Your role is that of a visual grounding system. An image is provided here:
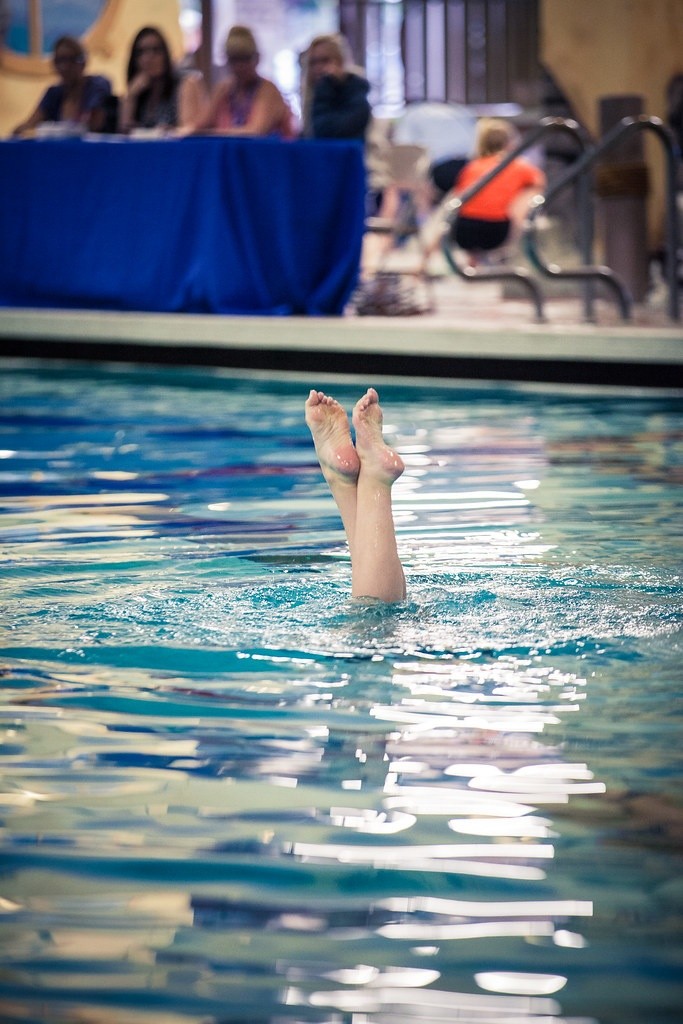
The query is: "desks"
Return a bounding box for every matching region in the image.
[0,133,369,318]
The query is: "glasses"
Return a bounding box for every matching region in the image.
[227,52,256,62]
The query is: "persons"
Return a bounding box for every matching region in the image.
[12,23,373,143]
[305,387,406,797]
[420,117,547,269]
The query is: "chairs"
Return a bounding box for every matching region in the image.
[366,145,426,278]
